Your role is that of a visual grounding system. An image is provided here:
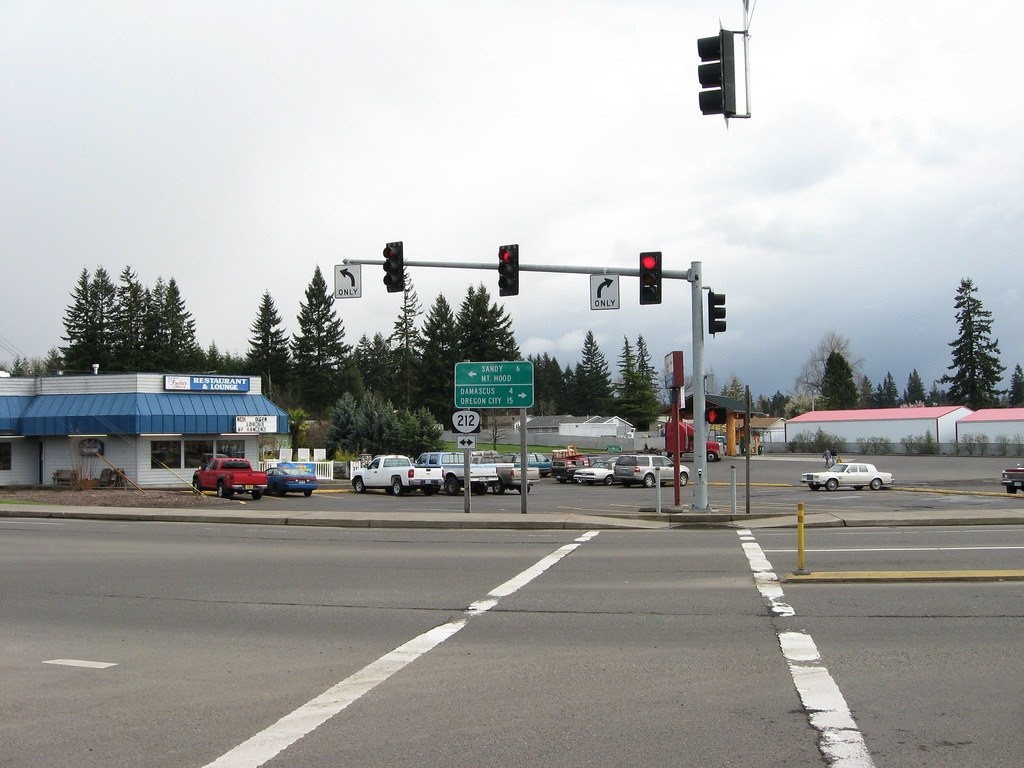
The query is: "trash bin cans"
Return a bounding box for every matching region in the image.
[741,447,746,454]
[607,445,623,453]
[758,445,763,455]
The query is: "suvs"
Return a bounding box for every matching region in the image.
[500,452,552,476]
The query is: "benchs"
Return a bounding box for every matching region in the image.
[52,469,78,486]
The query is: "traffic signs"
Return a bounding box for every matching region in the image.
[590,273,620,311]
[454,361,535,408]
[333,264,362,298]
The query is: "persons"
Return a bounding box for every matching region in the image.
[823,446,837,468]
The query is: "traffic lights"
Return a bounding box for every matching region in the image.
[639,251,662,305]
[707,292,727,334]
[697,30,737,116]
[382,241,404,293]
[498,244,519,297]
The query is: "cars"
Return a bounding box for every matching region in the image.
[573,461,617,486]
[613,454,690,488]
[1001,463,1024,494]
[265,467,318,497]
[800,463,893,492]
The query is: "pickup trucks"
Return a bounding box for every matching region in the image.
[412,452,498,496]
[188,453,230,467]
[351,454,445,497]
[193,458,268,501]
[551,456,606,484]
[471,451,541,495]
